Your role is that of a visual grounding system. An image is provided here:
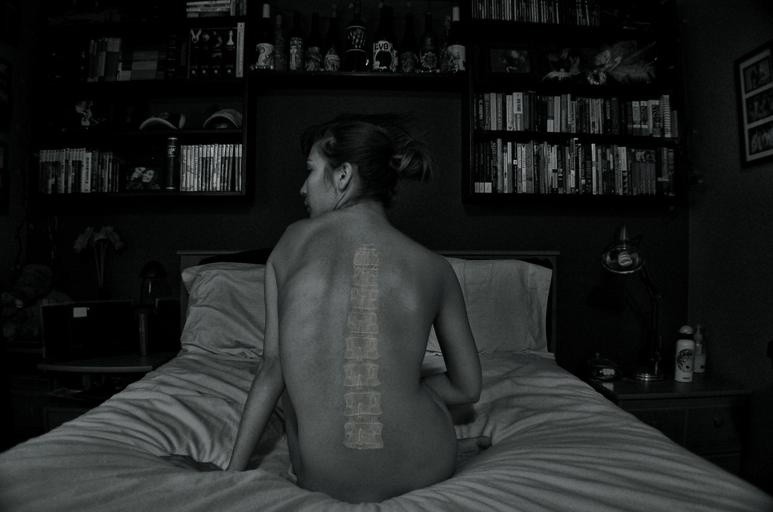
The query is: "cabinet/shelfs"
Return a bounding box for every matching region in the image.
[0,1,249,200]
[4,340,49,428]
[460,1,691,217]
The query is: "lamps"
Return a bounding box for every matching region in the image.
[599,224,665,383]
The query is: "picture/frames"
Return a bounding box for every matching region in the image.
[733,44,773,168]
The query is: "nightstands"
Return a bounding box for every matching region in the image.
[36,355,154,433]
[561,360,751,478]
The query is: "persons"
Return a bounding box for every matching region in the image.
[225,108,493,505]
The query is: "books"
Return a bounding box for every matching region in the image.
[474,88,680,197]
[33,146,121,194]
[87,37,122,83]
[179,144,242,193]
[470,0,592,28]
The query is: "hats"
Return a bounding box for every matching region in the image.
[202,108,242,129]
[140,109,186,130]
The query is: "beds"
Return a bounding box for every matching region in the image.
[0,248,772,512]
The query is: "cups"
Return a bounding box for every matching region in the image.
[287,36,304,69]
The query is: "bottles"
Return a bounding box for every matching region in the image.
[399,5,467,73]
[303,46,322,71]
[672,325,706,382]
[323,49,340,72]
[273,15,287,70]
[253,3,274,68]
